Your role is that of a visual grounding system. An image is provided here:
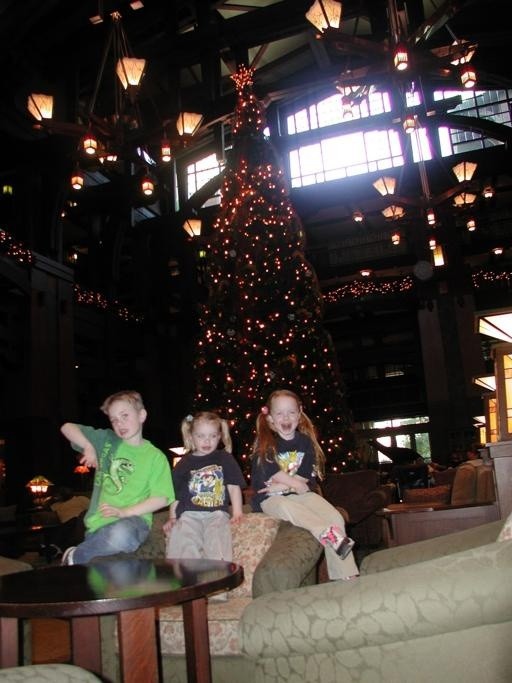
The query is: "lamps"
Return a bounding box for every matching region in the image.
[471,304,512,445]
[25,475,55,507]
[350,111,505,267]
[303,0,477,135]
[28,10,204,197]
[183,213,203,245]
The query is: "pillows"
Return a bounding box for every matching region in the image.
[158,513,281,593]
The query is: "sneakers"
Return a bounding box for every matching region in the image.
[60,546,77,567]
[319,527,356,561]
[208,592,229,603]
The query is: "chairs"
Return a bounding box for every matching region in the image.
[240,514,509,680]
[110,511,330,658]
[331,470,389,543]
[377,461,495,548]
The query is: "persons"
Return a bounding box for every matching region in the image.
[60,391,175,566]
[428,452,463,488]
[252,389,359,580]
[163,411,247,601]
